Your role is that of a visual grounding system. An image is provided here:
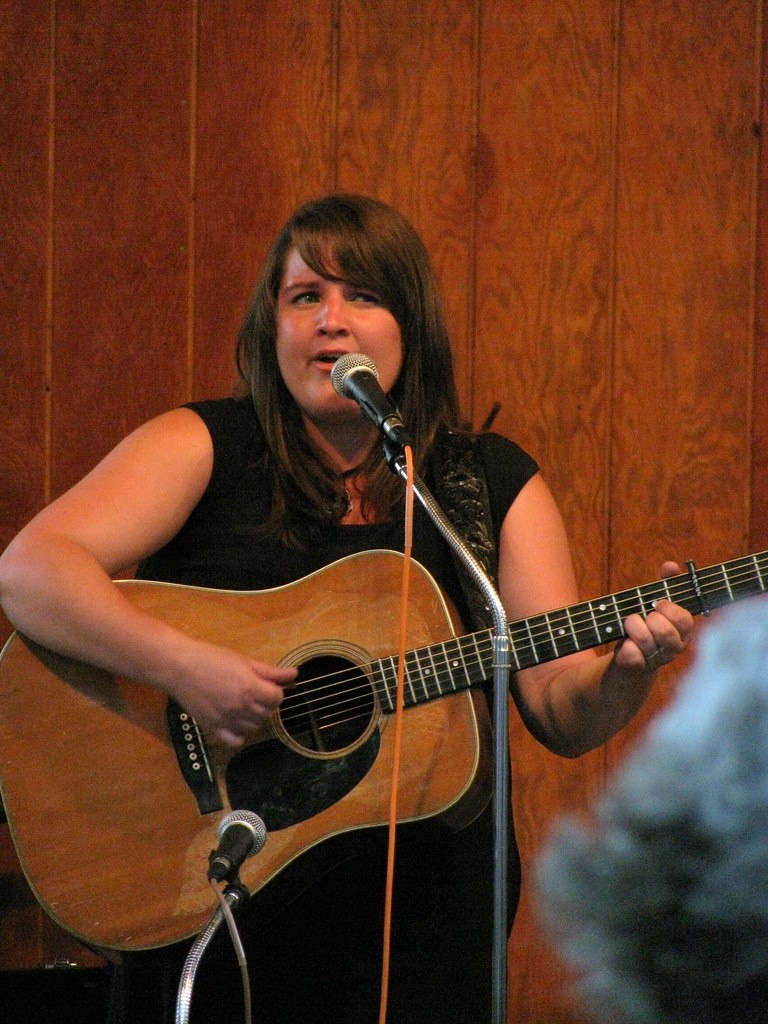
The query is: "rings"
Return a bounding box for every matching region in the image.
[645,651,659,659]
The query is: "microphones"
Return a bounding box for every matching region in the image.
[207,809,267,883]
[329,352,412,449]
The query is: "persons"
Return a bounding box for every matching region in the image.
[535,595,768,1023]
[1,191,694,1024]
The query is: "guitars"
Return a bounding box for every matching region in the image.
[0,549,768,955]
